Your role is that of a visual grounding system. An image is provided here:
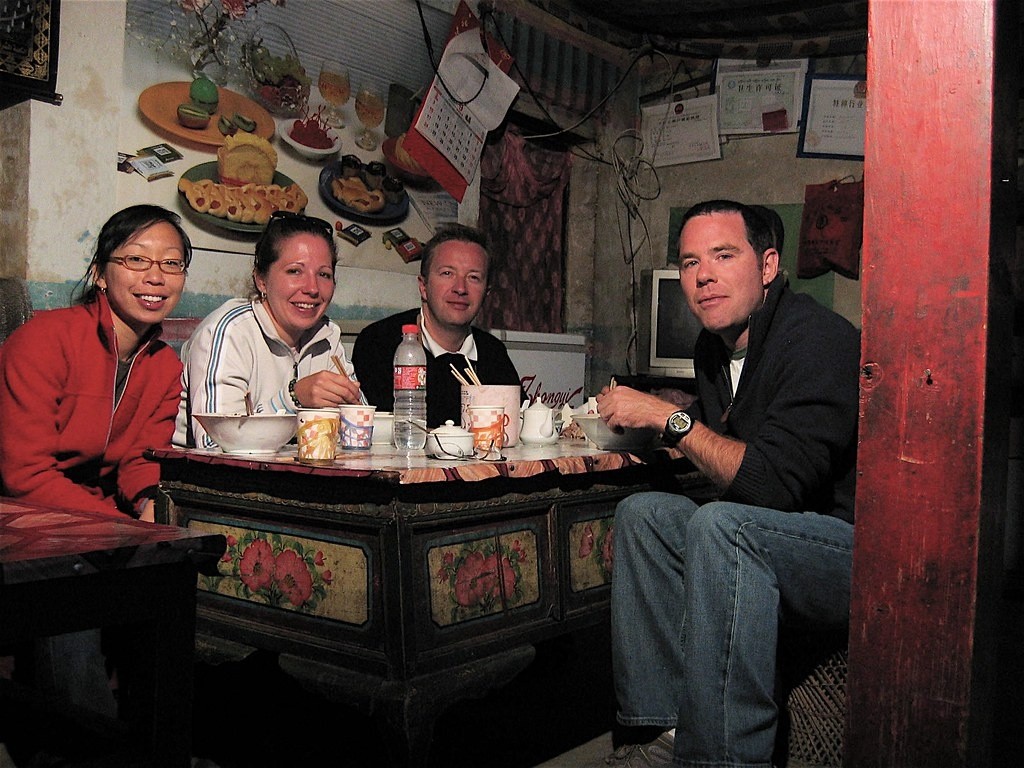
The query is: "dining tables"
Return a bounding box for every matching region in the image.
[138,438,725,768]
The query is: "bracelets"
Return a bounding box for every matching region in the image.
[289,378,300,407]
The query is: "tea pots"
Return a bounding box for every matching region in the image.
[519,397,558,445]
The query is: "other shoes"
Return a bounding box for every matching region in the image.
[584,729,676,768]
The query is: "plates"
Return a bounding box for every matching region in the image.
[382,138,434,180]
[177,160,306,232]
[319,160,409,221]
[139,82,276,145]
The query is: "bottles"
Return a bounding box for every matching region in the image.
[393,324,427,449]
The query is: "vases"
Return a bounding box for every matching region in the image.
[187,10,232,87]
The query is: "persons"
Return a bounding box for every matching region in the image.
[352,223,532,430]
[169,211,368,477]
[594,200,862,768]
[0,206,194,720]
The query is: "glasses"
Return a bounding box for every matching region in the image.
[255,210,335,253]
[103,254,189,275]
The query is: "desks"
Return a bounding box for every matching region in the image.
[0,497,225,768]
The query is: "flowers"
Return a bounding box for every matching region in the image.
[125,1,308,110]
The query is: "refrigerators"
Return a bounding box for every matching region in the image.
[329,319,587,411]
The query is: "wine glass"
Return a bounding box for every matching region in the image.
[353,82,385,152]
[318,60,351,128]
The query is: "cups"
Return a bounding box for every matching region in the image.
[467,405,505,450]
[296,408,341,465]
[460,385,521,447]
[338,405,376,450]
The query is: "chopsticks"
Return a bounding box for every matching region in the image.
[331,355,349,379]
[449,356,481,386]
[244,390,255,416]
[610,377,616,391]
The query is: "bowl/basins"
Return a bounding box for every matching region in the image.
[371,411,395,445]
[570,413,657,451]
[426,420,475,460]
[278,118,343,159]
[190,413,298,454]
[554,420,565,434]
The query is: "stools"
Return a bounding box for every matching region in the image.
[779,625,849,768]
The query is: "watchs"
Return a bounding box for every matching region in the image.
[663,409,697,446]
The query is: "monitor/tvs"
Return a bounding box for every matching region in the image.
[649,268,705,379]
